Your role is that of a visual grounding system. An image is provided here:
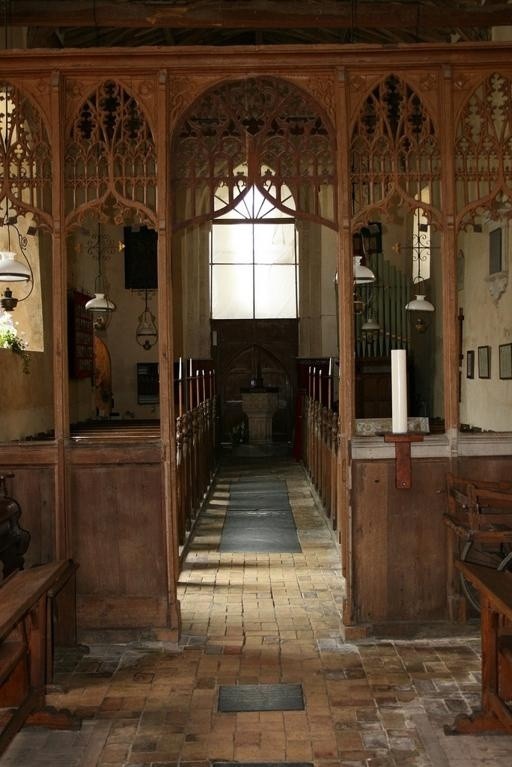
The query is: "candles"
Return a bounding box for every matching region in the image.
[389,349,410,435]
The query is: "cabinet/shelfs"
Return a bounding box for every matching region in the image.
[67,286,97,379]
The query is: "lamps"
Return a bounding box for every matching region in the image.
[335,210,436,336]
[85,223,116,318]
[0,91,33,300]
[135,291,159,350]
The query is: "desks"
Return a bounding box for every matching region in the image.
[443,560,511,739]
[2,558,85,765]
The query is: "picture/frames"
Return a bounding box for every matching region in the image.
[464,342,512,380]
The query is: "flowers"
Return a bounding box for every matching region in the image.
[0,314,33,375]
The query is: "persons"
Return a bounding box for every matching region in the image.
[354,366,371,418]
